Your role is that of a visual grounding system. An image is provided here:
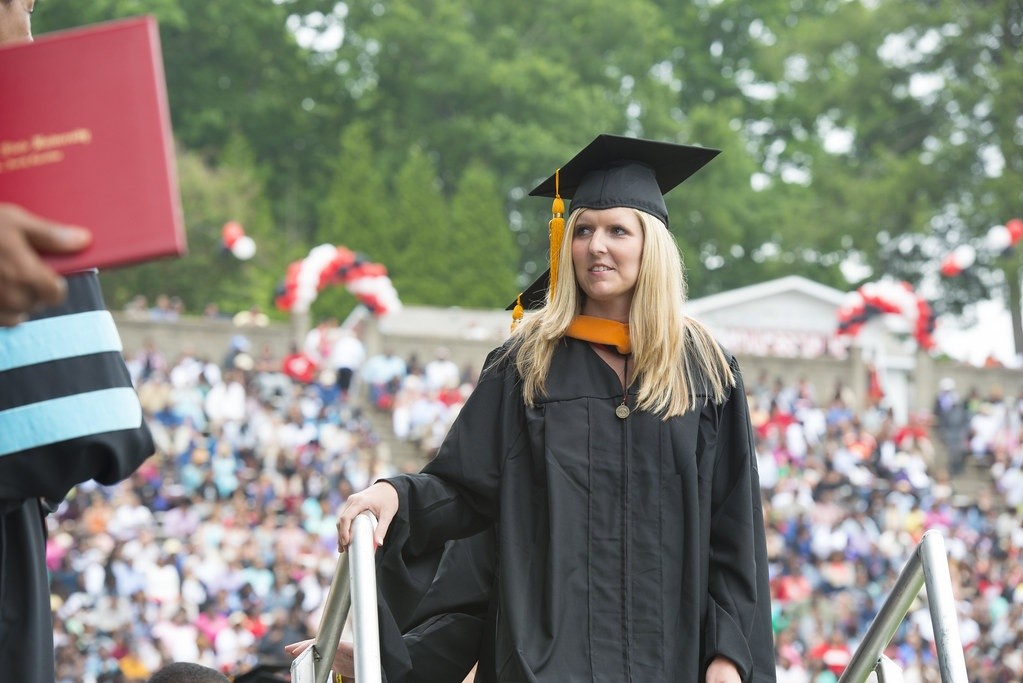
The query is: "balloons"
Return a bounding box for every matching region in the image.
[918,218,1023,300]
[272,245,402,317]
[221,222,256,261]
[835,276,934,350]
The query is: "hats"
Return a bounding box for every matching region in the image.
[528,133,724,313]
[506,264,552,335]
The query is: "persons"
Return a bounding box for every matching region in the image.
[0,0,157,683]
[44,296,483,683]
[738,376,1023,683]
[338,133,776,683]
